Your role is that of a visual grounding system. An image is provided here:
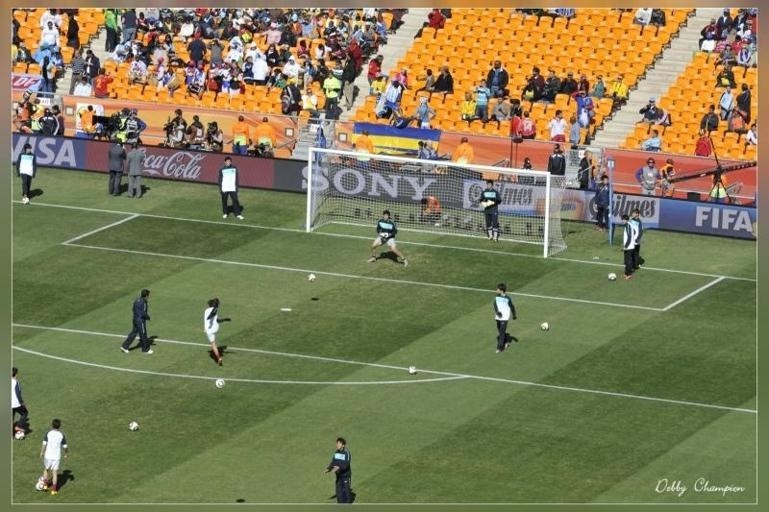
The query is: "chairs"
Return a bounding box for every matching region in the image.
[12,9,759,160]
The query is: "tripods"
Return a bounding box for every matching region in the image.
[704,179,731,204]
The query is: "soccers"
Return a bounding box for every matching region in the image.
[308,273,315,282]
[409,366,416,373]
[129,421,138,431]
[23,198,30,205]
[216,379,225,388]
[608,273,616,281]
[15,431,24,440]
[36,482,45,491]
[541,322,548,330]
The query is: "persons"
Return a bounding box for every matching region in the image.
[620,215,635,279]
[120,288,154,354]
[367,209,409,267]
[493,282,517,353]
[630,208,646,270]
[40,418,69,494]
[12,8,758,243]
[203,297,232,367]
[324,437,353,503]
[12,367,29,432]
